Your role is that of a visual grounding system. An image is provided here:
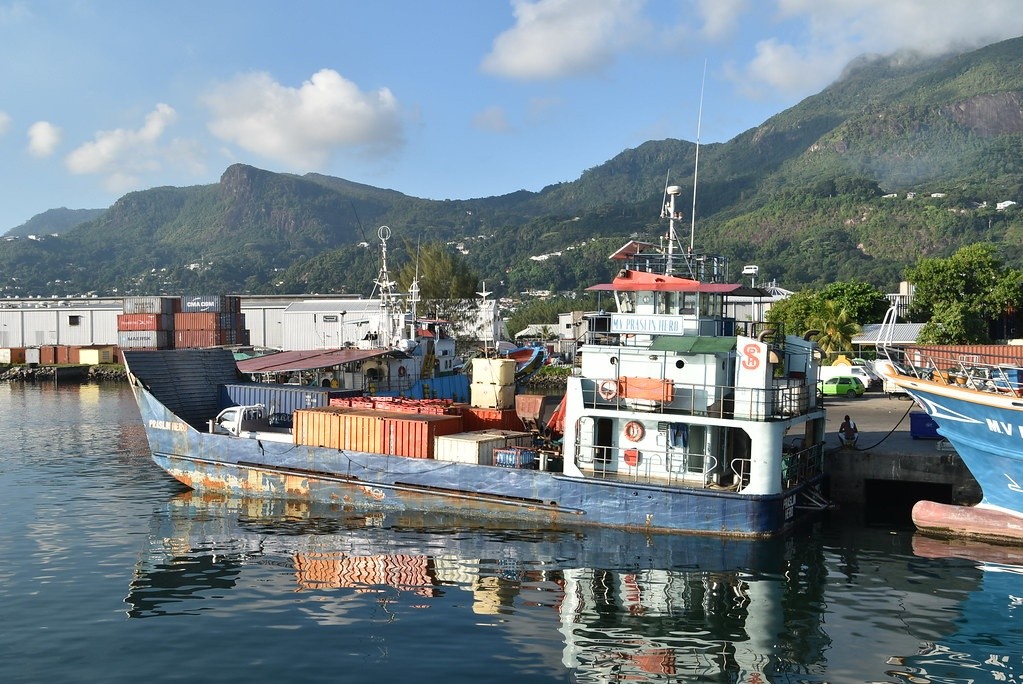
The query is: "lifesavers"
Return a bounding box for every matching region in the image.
[624,422,644,440]
[600,380,618,400]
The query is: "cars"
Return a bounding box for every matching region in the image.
[816,364,881,399]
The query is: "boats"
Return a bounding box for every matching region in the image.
[122,170,837,541]
[862,293,1022,517]
[240,224,547,405]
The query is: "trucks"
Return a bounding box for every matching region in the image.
[205,403,293,444]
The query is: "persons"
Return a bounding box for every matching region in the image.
[838,415,859,448]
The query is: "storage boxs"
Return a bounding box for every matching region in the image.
[903,344,1022,392]
[0,293,546,470]
